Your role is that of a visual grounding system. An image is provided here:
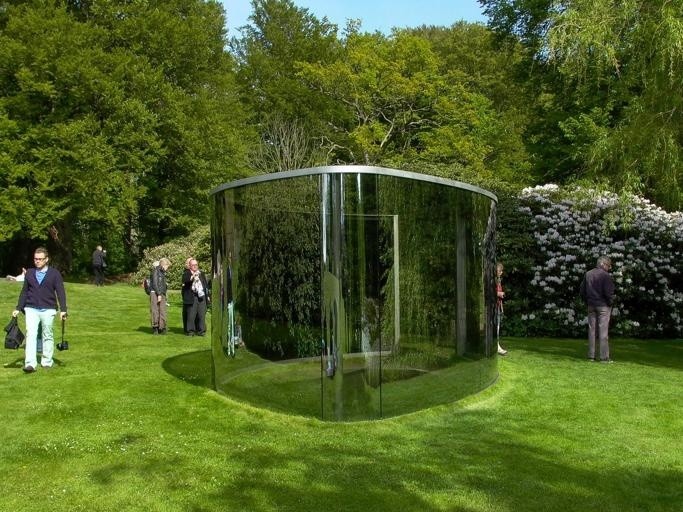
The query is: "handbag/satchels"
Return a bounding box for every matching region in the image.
[5,325,25,349]
[144,279,150,294]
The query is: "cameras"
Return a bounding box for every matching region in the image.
[57,340,69,351]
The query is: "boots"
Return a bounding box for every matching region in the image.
[154,327,167,335]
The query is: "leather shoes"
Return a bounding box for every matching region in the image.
[23,366,36,372]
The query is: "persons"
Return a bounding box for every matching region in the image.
[211,249,245,358]
[148,257,171,335]
[182,259,211,337]
[10,246,66,373]
[579,255,617,365]
[486,262,508,355]
[180,257,202,333]
[91,245,107,287]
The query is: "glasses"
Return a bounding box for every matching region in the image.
[34,258,46,261]
